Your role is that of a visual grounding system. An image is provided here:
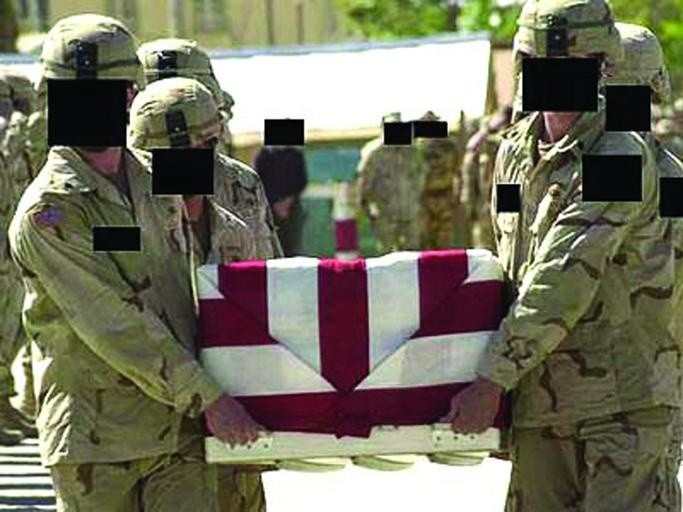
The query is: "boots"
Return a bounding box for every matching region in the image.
[0,395,38,444]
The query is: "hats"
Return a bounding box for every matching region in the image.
[380,111,402,123]
[421,111,437,121]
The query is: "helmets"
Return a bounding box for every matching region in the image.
[129,40,234,150]
[0,75,34,103]
[511,0,671,105]
[39,13,146,84]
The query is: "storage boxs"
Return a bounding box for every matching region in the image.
[196,247,514,464]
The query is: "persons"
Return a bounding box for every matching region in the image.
[598,22,681,268]
[127,78,268,512]
[437,1,680,511]
[0,39,306,448]
[7,13,268,512]
[359,106,509,255]
[650,100,682,164]
